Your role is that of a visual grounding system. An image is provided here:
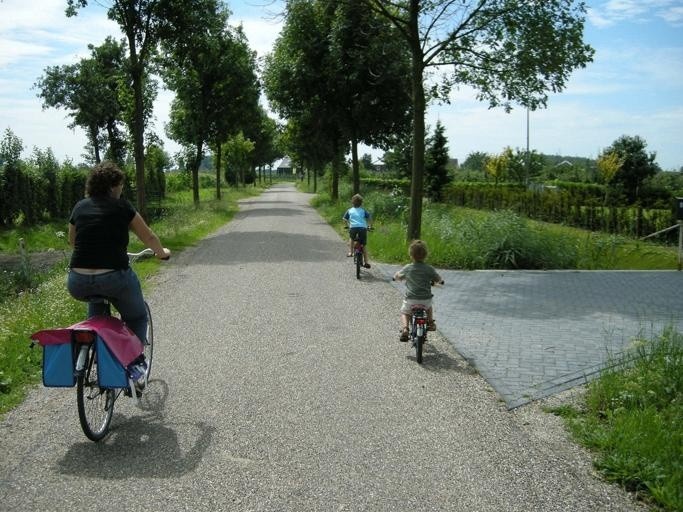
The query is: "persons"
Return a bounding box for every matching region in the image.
[67,159,170,389]
[343,193,371,270]
[395,240,442,341]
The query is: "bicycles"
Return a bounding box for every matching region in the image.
[391,276,445,364]
[76,249,170,443]
[344,226,375,279]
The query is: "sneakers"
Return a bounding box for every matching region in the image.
[130,360,147,382]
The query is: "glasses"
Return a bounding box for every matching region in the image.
[119,183,123,188]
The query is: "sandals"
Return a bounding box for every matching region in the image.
[347,251,353,257]
[364,263,370,268]
[427,321,436,331]
[399,327,410,342]
